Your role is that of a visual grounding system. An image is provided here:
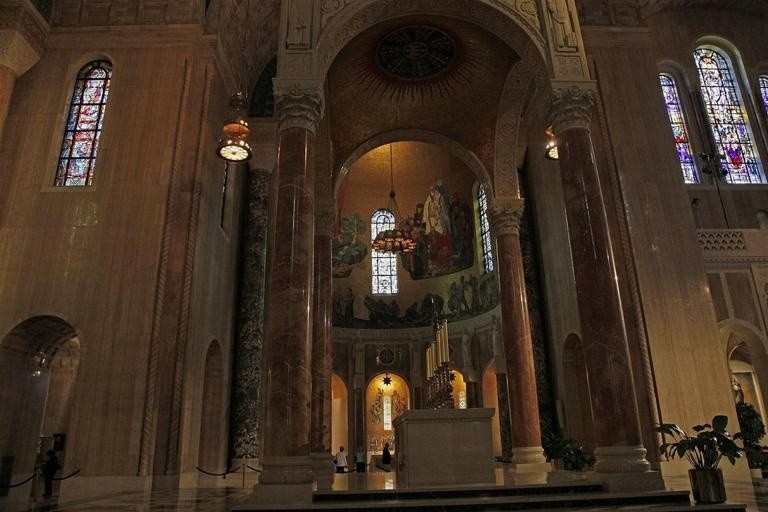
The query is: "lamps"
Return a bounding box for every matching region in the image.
[545,120,560,162]
[219,0,253,165]
[369,144,417,256]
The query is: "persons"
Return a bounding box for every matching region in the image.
[398,175,476,282]
[381,442,392,472]
[41,449,58,498]
[353,446,366,473]
[334,446,348,473]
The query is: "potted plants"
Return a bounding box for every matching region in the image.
[653,399,767,503]
[541,417,596,479]
